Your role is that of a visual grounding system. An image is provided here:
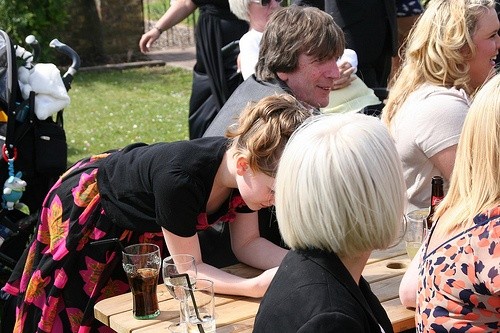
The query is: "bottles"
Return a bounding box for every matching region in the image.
[427,175,445,234]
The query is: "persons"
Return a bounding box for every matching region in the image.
[229,0,384,119]
[201,4,345,269]
[254,113,408,333]
[296,0,398,88]
[379,0,500,212]
[140,0,229,138]
[399,74,500,333]
[0,93,312,333]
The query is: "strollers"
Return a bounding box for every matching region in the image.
[0,28,82,333]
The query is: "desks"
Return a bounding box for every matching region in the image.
[93,226,416,332]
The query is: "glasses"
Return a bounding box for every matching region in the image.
[250,0,282,7]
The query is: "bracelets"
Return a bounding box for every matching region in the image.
[153,26,162,34]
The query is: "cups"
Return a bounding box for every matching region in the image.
[183,279,216,333]
[122,243,162,319]
[404,208,430,260]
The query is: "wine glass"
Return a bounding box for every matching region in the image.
[162,254,198,333]
